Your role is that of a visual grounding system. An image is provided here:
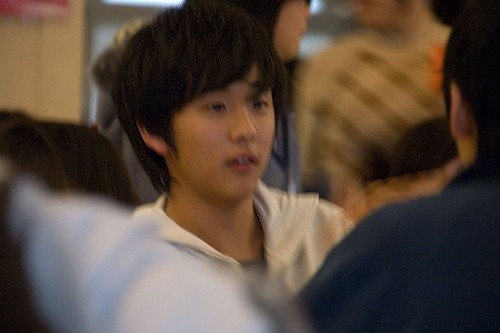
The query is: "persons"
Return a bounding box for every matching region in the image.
[0,0,461,225]
[287,0,500,333]
[108,0,358,333]
[0,157,270,333]
